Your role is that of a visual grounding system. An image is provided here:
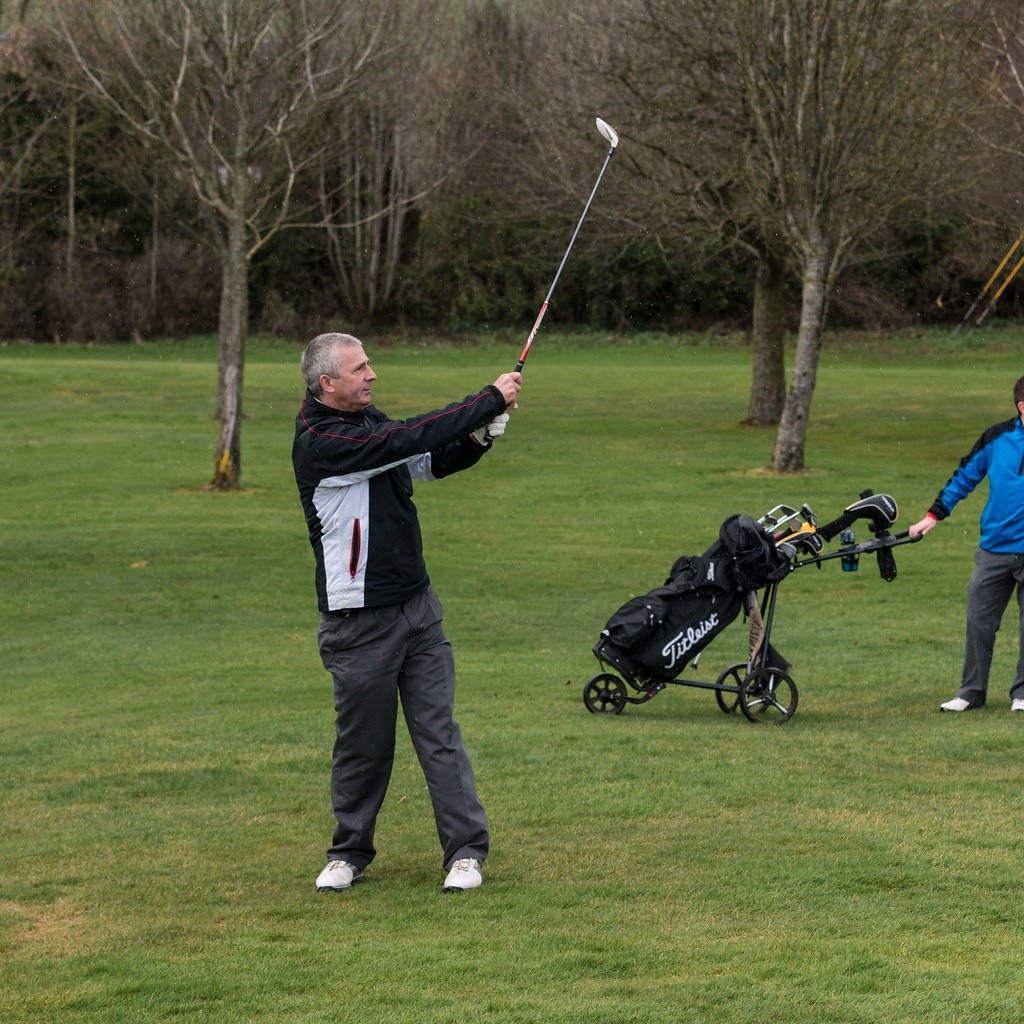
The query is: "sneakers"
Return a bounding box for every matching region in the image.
[442,857,482,891]
[940,697,977,711]
[1011,698,1024,711]
[315,858,363,890]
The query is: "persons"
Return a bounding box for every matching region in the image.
[293,333,523,894]
[908,375,1024,712]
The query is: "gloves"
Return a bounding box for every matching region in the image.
[472,403,518,446]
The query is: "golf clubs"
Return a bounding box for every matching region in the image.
[484,116,620,441]
[755,503,824,563]
